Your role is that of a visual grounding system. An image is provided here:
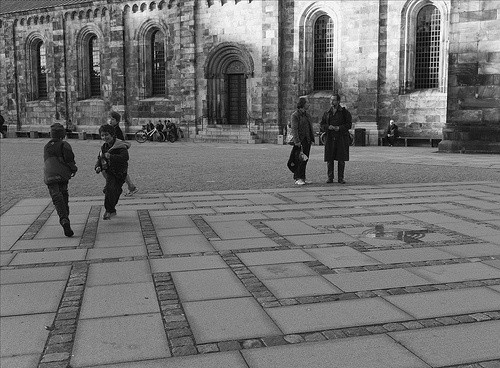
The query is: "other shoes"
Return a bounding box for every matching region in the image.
[326,179,333,182]
[125,188,139,196]
[102,211,116,220]
[338,180,345,184]
[62,221,74,237]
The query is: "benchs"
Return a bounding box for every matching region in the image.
[15,130,50,138]
[65,132,100,140]
[126,132,148,141]
[380,137,442,148]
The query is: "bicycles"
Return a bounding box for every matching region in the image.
[319,131,355,146]
[135,125,165,143]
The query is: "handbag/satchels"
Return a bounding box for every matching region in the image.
[287,145,303,173]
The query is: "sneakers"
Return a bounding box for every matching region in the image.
[295,179,305,184]
[305,180,312,183]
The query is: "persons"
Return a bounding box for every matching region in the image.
[386,120,399,147]
[320,94,352,184]
[291,97,315,185]
[145,119,181,142]
[94,124,131,220]
[44,123,78,238]
[108,111,139,196]
